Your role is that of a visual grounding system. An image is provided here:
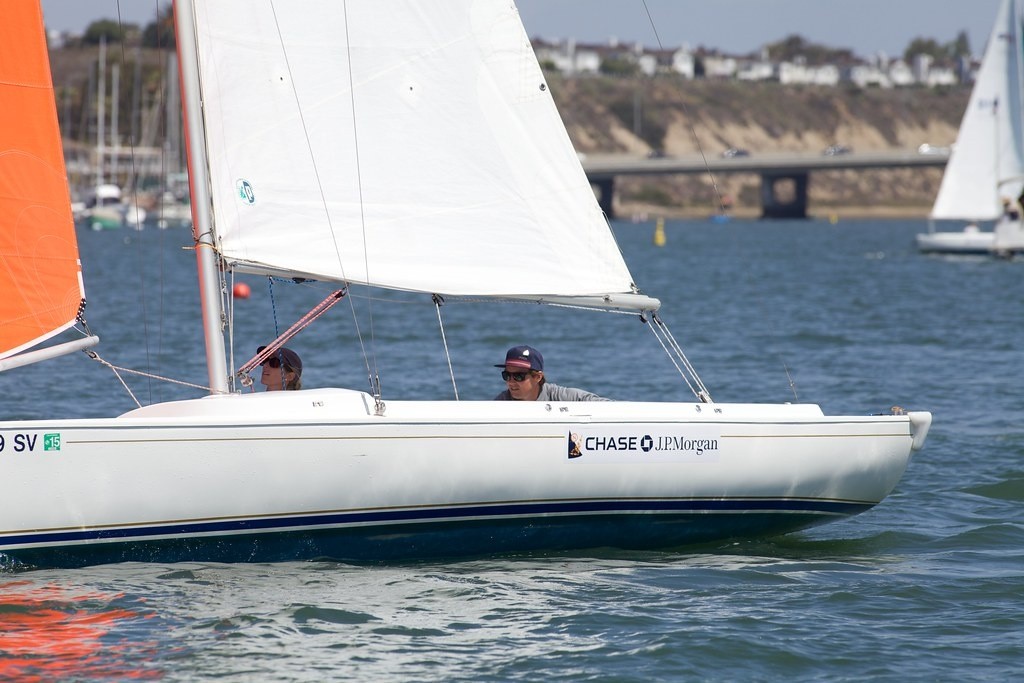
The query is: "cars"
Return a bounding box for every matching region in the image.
[645,148,673,159]
[719,145,751,157]
[821,142,854,157]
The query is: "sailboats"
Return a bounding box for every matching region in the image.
[917,0,1024,257]
[0,0,931,569]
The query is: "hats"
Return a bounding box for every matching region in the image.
[257,346,302,378]
[493,345,544,372]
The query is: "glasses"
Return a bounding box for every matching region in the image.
[259,354,281,368]
[501,371,527,382]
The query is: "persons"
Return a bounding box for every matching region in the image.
[256,346,302,391]
[492,345,614,401]
